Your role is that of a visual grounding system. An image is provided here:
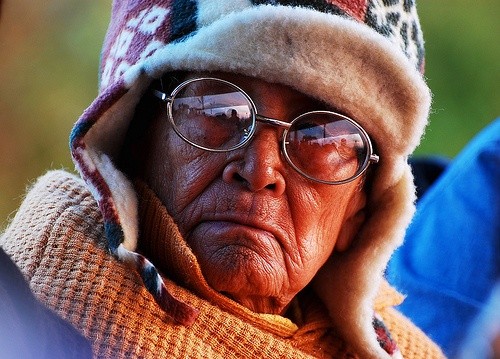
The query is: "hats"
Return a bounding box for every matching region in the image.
[69,2,434,359]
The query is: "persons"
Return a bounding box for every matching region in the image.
[0,1,447,359]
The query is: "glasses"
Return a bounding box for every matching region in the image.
[152,78,380,185]
